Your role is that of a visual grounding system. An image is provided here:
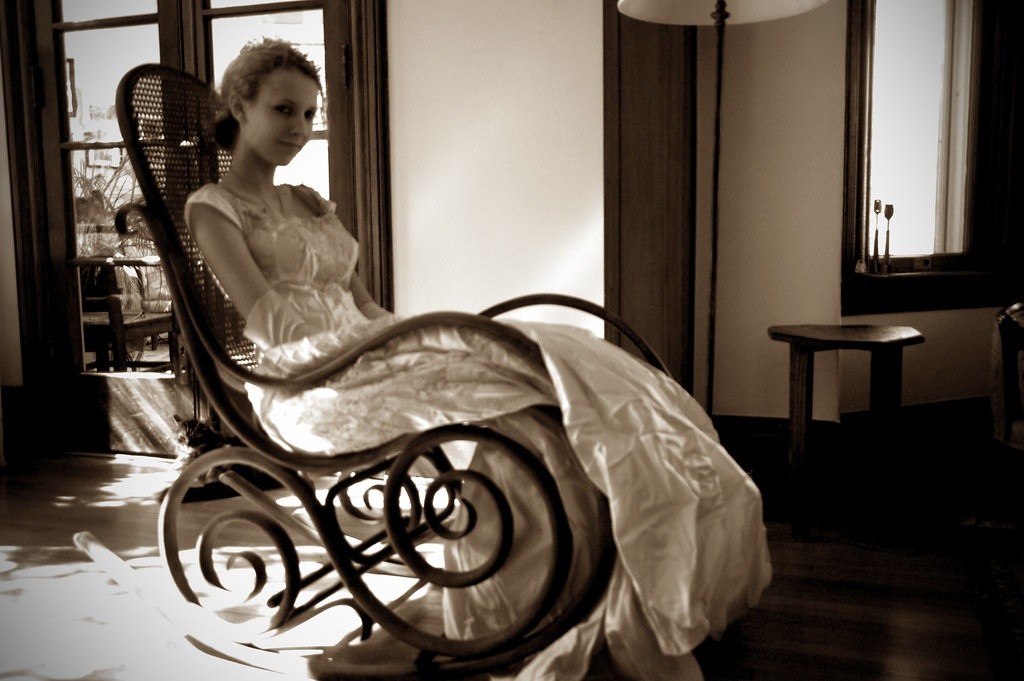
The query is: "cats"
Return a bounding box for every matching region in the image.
[153,414,286,506]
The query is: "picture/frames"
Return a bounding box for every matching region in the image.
[85,146,124,170]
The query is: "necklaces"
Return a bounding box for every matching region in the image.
[226,165,288,223]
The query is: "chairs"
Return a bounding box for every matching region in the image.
[73,63,676,681]
[73,254,185,373]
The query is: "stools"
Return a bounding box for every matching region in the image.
[767,325,925,543]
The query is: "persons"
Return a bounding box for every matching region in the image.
[184,37,773,681]
